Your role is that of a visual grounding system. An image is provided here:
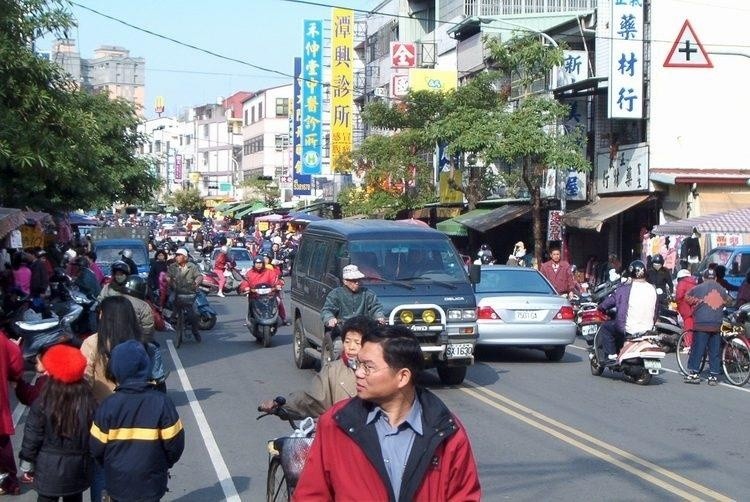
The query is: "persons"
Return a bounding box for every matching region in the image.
[291,327,482,502]
[509,241,750,386]
[0,215,103,327]
[89,339,185,502]
[258,313,379,420]
[102,214,303,276]
[1,296,142,493]
[91,248,146,311]
[360,247,438,279]
[20,345,100,502]
[320,264,389,358]
[148,249,202,341]
[214,244,228,298]
[239,256,292,326]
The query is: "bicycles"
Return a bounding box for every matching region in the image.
[674,306,749,389]
[251,395,318,501]
[170,280,199,348]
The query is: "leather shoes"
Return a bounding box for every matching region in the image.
[599,360,616,365]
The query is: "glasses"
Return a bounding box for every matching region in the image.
[356,360,388,372]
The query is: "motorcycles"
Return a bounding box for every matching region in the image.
[7,280,104,368]
[230,266,289,347]
[571,274,682,384]
[152,280,220,332]
[196,258,250,297]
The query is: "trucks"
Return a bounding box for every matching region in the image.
[693,244,749,308]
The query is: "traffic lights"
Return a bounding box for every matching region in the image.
[256,175,276,182]
[208,184,220,189]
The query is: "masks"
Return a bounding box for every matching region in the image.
[653,264,662,270]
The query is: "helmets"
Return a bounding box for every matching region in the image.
[123,249,132,259]
[119,275,145,298]
[253,256,263,262]
[630,261,644,278]
[111,260,129,273]
[652,254,663,262]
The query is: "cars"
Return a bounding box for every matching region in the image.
[465,262,578,360]
[75,208,292,282]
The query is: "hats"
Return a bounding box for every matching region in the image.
[703,268,716,279]
[677,269,691,279]
[176,249,187,256]
[343,264,364,279]
[41,344,87,383]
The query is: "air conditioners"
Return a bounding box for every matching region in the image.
[464,150,488,167]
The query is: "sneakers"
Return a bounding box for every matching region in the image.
[708,376,717,385]
[685,374,699,383]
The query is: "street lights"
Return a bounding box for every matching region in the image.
[477,14,567,260]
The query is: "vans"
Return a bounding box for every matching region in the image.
[283,219,479,385]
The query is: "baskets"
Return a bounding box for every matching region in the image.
[280,437,314,485]
[175,292,199,305]
